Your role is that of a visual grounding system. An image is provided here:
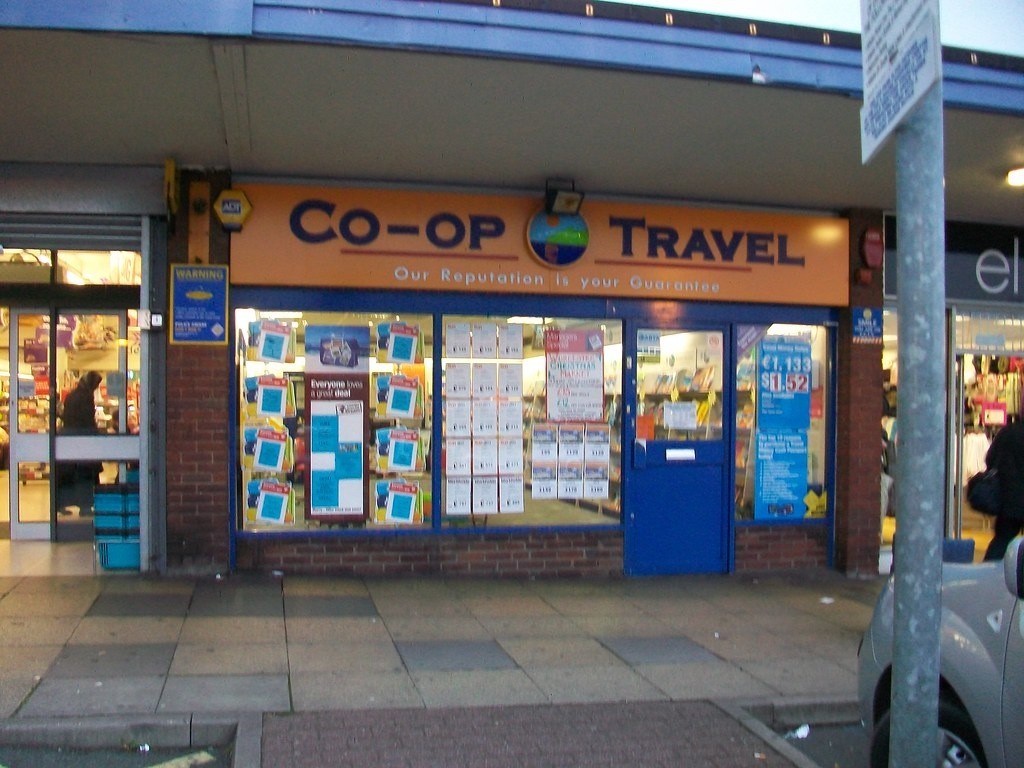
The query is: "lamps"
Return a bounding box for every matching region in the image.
[545,188,585,216]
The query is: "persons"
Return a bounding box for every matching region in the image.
[109,410,136,470]
[54,370,104,516]
[966,418,1024,563]
[879,385,898,473]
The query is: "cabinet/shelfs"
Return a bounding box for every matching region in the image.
[429,365,756,516]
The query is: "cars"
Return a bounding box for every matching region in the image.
[855,528,1024,768]
